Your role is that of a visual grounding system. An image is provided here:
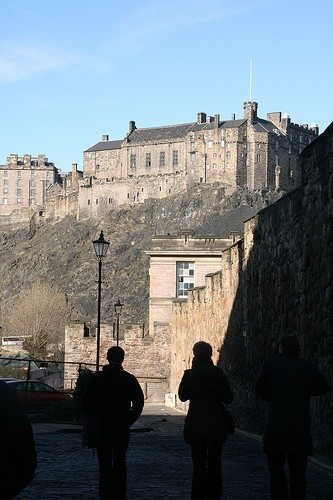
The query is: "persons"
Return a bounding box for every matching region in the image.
[76,347,144,500]
[255,336,330,500]
[0,378,40,500]
[178,341,236,500]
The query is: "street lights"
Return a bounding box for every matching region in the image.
[91,228,111,370]
[113,299,124,347]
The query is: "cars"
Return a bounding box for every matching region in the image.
[0,377,75,418]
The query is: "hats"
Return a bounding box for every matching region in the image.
[193,341,212,357]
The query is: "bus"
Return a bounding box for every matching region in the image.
[1,335,36,347]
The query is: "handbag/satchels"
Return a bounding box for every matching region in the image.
[209,411,232,446]
